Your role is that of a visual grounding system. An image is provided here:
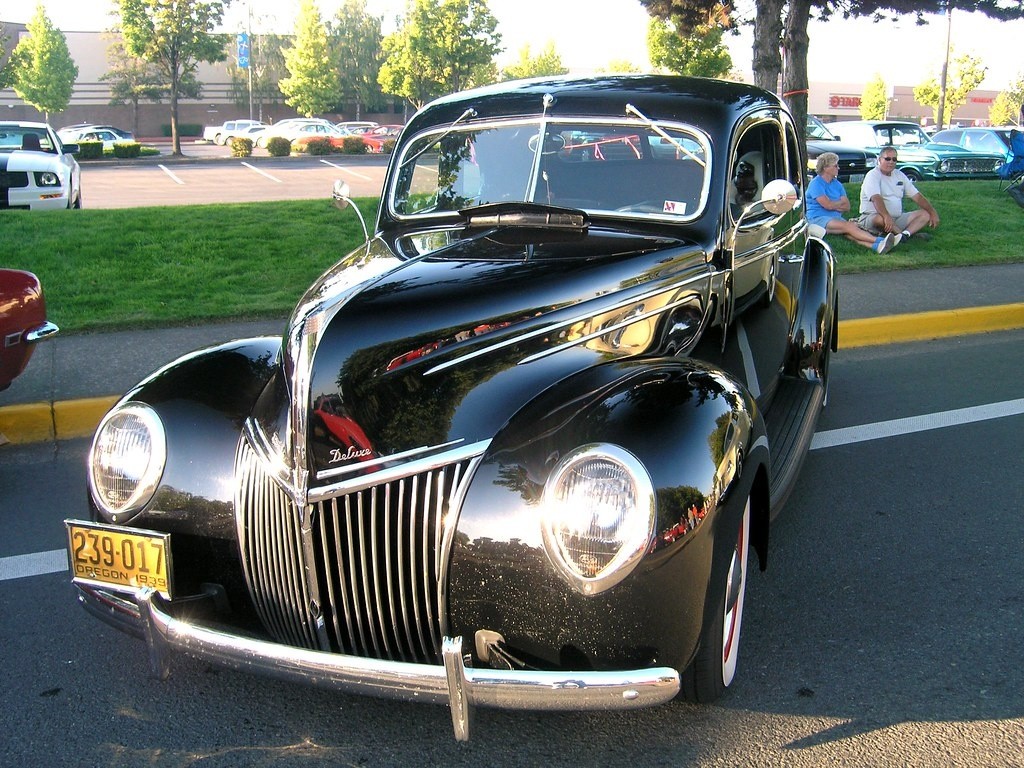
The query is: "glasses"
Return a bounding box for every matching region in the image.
[883,156,897,162]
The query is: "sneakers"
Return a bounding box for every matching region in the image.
[877,233,902,255]
[900,232,909,243]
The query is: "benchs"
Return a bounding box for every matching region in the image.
[524,158,706,216]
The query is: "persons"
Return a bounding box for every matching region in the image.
[805,152,894,255]
[858,147,939,243]
[685,504,700,530]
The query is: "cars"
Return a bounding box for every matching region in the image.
[0,267,60,392]
[62,73,841,743]
[55,124,135,150]
[0,121,81,211]
[806,114,1024,183]
[202,118,405,153]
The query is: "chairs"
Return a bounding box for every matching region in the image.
[995,129,1024,191]
